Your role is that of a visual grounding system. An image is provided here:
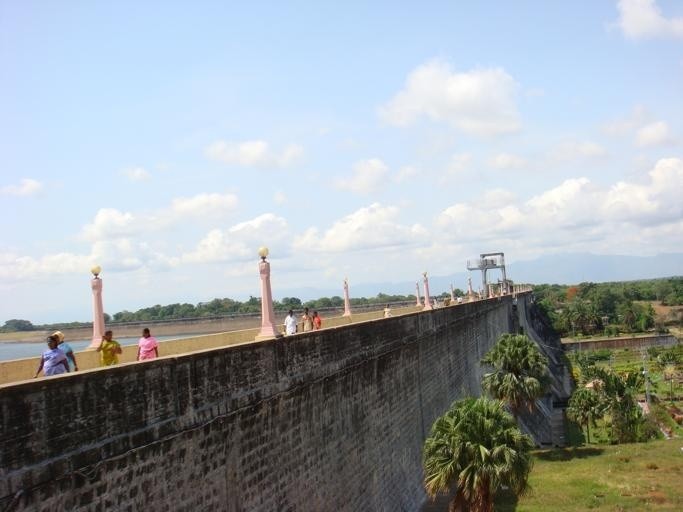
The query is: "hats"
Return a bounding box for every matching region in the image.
[52,331,64,343]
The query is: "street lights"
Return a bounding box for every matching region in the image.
[422,270,433,310]
[89,264,105,347]
[642,355,650,401]
[415,281,420,305]
[450,277,526,301]
[257,247,277,336]
[671,374,674,403]
[343,275,352,315]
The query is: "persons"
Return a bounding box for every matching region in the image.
[96,331,122,367]
[383,303,391,318]
[301,308,313,332]
[33,331,78,378]
[434,295,463,306]
[284,310,298,335]
[136,328,158,361]
[312,312,321,329]
